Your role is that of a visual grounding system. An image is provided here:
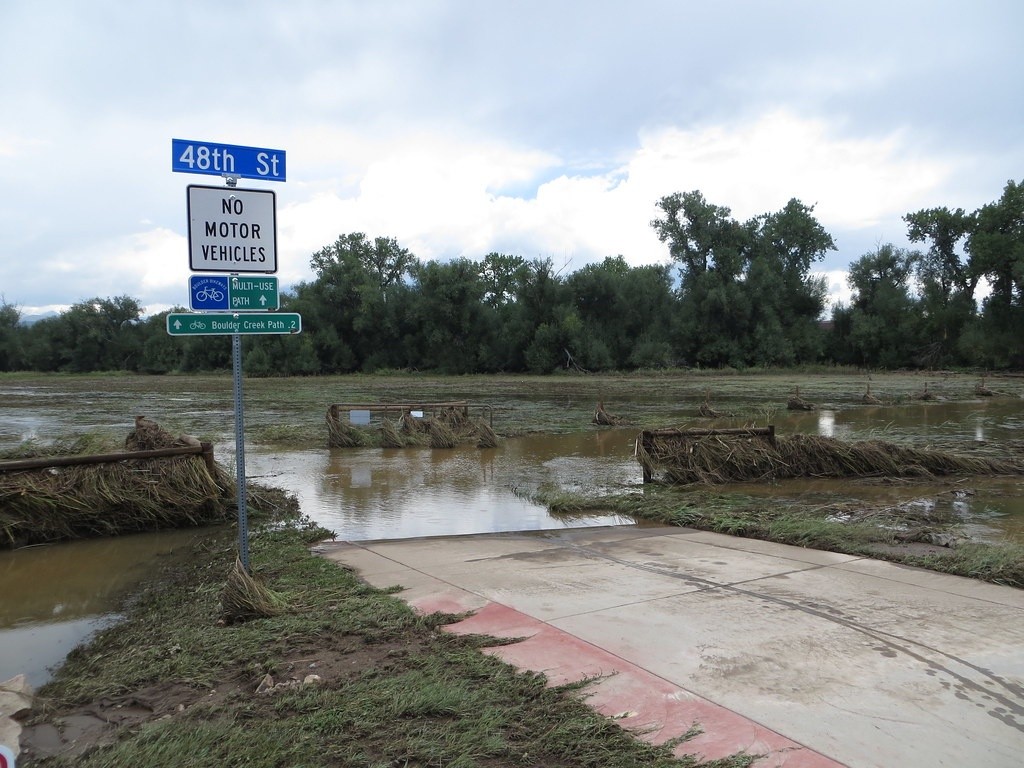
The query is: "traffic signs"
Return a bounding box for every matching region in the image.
[229,274,280,312]
[166,313,302,335]
